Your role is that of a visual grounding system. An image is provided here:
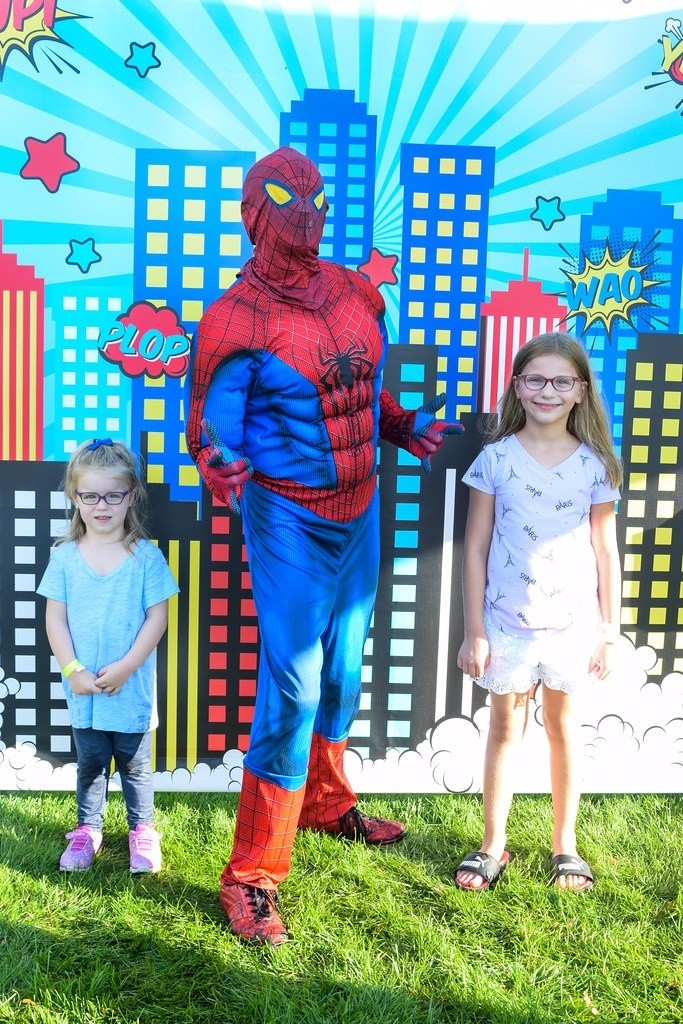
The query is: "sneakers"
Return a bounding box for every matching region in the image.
[128,824,162,874]
[320,805,408,845]
[60,825,102,870]
[218,882,288,945]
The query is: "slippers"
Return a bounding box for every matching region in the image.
[454,847,510,891]
[547,849,596,894]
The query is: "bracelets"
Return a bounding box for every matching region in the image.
[61,660,85,677]
[604,641,615,645]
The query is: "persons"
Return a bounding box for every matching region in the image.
[455,333,623,890]
[187,149,465,946]
[36,439,181,871]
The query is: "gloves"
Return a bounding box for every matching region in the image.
[410,393,465,472]
[196,419,253,514]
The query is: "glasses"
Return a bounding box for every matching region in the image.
[516,373,581,392]
[75,489,133,505]
